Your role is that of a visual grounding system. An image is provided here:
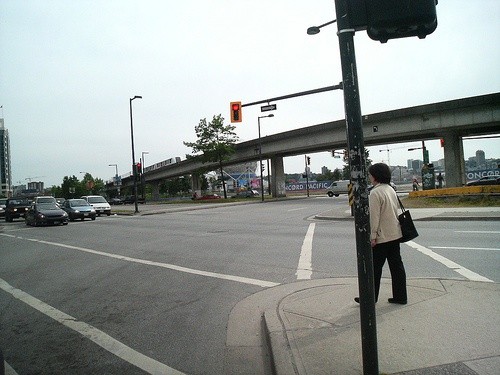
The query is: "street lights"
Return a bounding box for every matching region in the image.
[258,113,274,201]
[129,96,143,213]
[408,147,425,166]
[142,151,149,204]
[109,164,120,198]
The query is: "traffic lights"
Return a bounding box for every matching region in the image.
[229,102,242,122]
[261,164,265,171]
[307,157,310,165]
[332,149,335,157]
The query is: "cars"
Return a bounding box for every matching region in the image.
[56,198,65,205]
[32,196,60,207]
[108,198,123,205]
[81,196,111,216]
[465,175,500,185]
[24,203,69,227]
[0,198,33,222]
[61,199,96,222]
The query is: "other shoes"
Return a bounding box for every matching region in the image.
[354,297,360,303]
[388,298,408,304]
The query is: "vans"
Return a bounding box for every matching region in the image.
[325,180,350,198]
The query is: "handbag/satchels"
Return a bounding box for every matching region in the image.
[398,210,418,243]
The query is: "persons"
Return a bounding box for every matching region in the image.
[436,173,444,189]
[354,163,407,305]
[413,179,420,191]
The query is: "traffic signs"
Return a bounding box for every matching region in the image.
[261,104,276,111]
